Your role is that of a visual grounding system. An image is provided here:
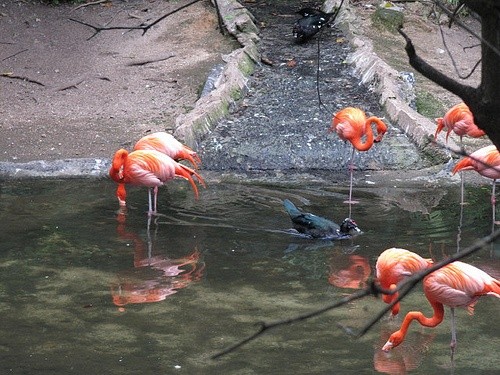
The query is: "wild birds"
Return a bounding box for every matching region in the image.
[381,260,500,352]
[111,206,206,306]
[373,247,434,317]
[373,317,438,375]
[432,102,486,146]
[329,202,371,290]
[108,132,206,211]
[450,226,500,281]
[327,107,388,204]
[451,144,500,225]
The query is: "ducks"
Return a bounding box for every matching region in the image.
[284,197,362,239]
[283,239,360,256]
